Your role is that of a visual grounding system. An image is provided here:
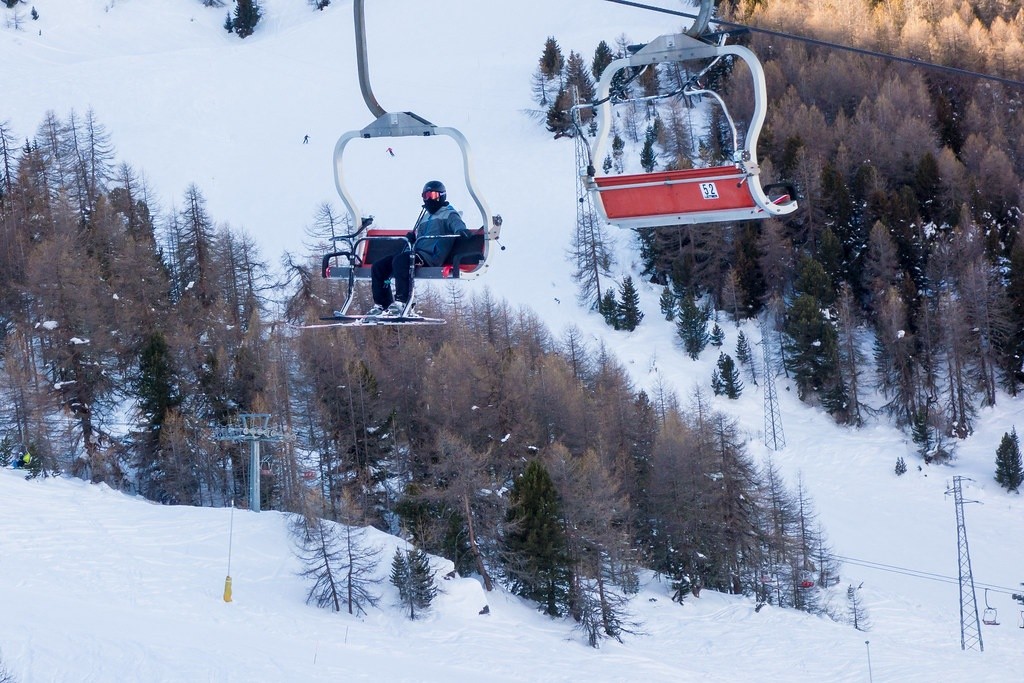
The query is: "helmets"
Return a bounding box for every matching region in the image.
[422,181,446,205]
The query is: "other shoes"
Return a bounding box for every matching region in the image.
[382,300,414,316]
[361,304,383,322]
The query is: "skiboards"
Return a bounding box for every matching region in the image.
[284,309,448,330]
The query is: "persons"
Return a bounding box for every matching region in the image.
[13,451,31,468]
[360,181,473,322]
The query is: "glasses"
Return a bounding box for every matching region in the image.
[423,191,440,200]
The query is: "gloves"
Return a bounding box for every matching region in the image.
[460,229,472,241]
[406,232,416,244]
